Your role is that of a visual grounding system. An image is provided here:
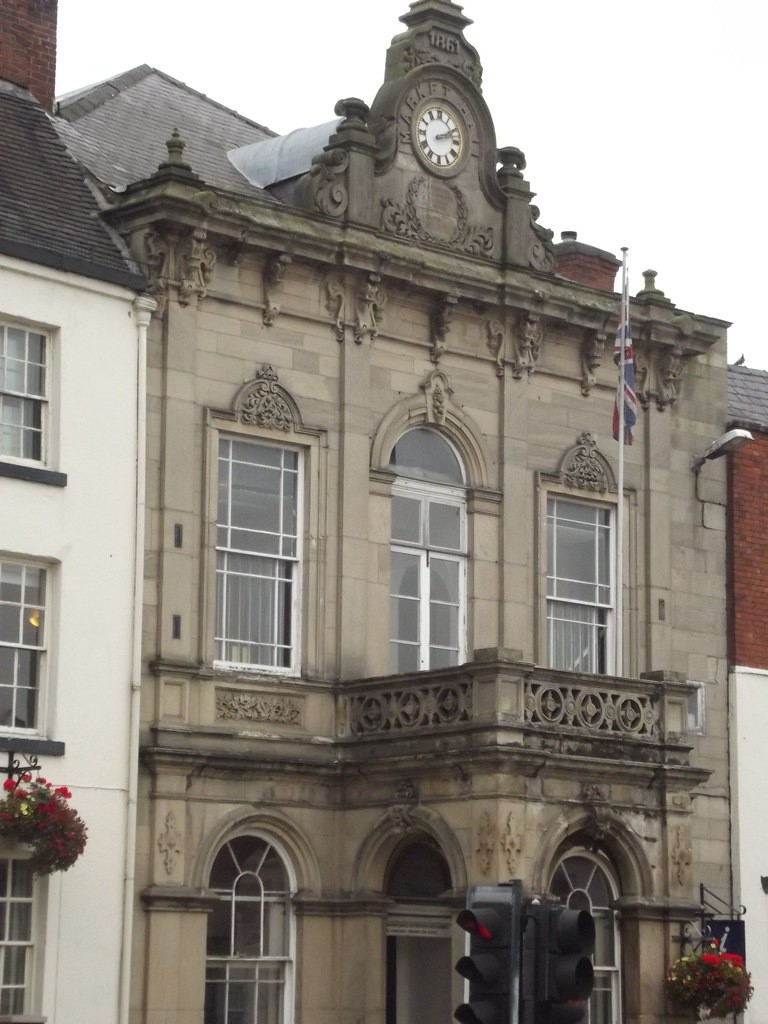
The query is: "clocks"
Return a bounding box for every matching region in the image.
[410,95,474,181]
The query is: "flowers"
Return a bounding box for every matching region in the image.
[0,775,88,880]
[664,938,755,1024]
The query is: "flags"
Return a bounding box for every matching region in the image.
[612,274,637,446]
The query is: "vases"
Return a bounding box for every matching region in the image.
[0,831,35,860]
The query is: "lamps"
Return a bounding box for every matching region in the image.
[691,430,754,476]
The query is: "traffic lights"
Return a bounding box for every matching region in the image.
[453,879,523,1024]
[536,902,596,1024]
